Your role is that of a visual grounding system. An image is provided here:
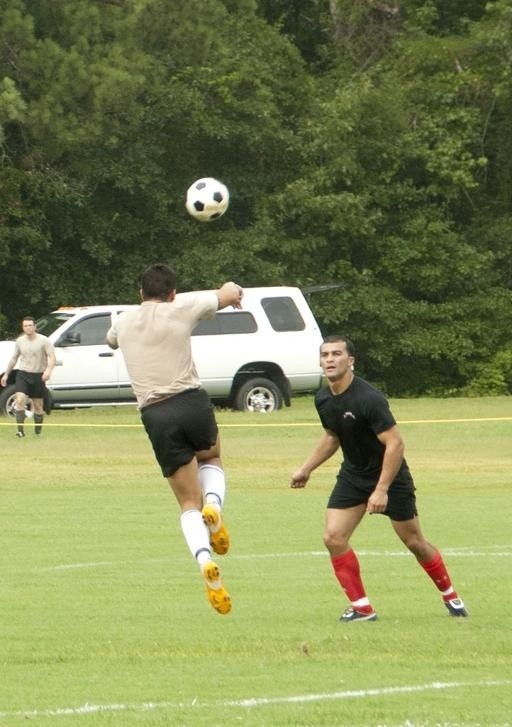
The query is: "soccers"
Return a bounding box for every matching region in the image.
[186,177,229,222]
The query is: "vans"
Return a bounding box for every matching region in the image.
[1,285,358,425]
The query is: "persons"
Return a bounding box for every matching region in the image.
[0,315,57,437]
[288,333,467,621]
[106,262,245,616]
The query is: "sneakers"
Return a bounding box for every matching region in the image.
[442,594,470,618]
[337,607,377,623]
[203,562,232,615]
[201,506,229,554]
[15,432,25,438]
